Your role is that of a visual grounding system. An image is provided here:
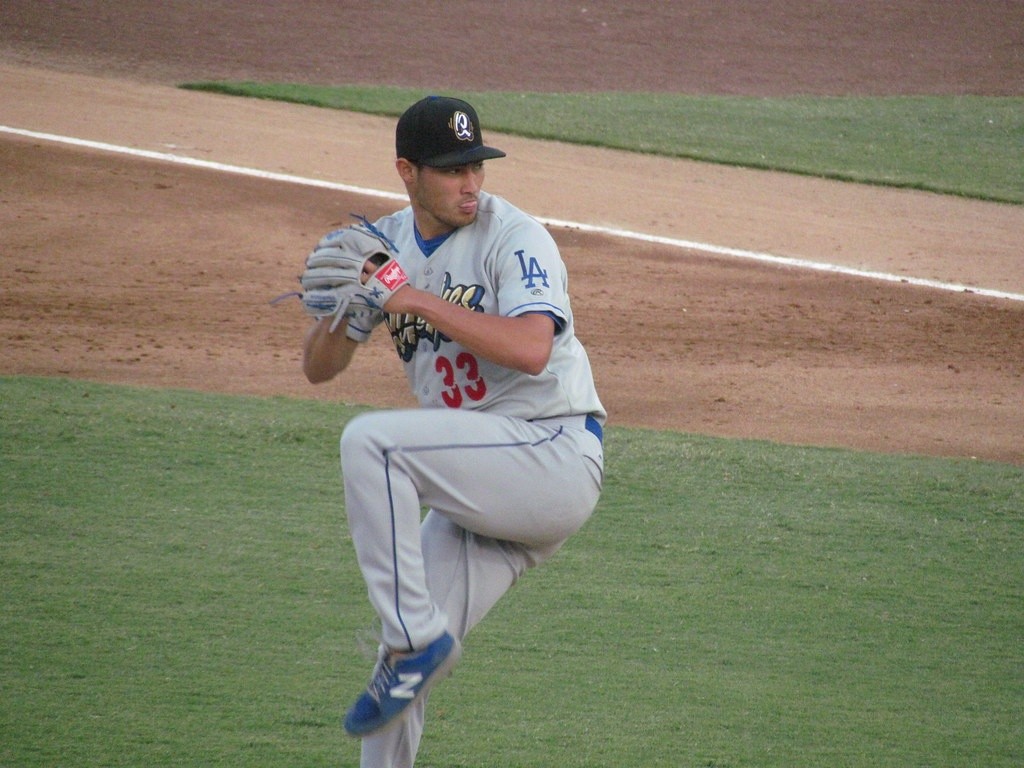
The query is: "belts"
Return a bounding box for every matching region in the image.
[585,414,602,443]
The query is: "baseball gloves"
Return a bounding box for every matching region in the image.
[295,225,409,343]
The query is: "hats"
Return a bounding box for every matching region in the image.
[396,96,506,166]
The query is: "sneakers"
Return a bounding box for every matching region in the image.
[345,630,461,737]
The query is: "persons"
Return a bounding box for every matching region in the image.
[298,96,608,768]
